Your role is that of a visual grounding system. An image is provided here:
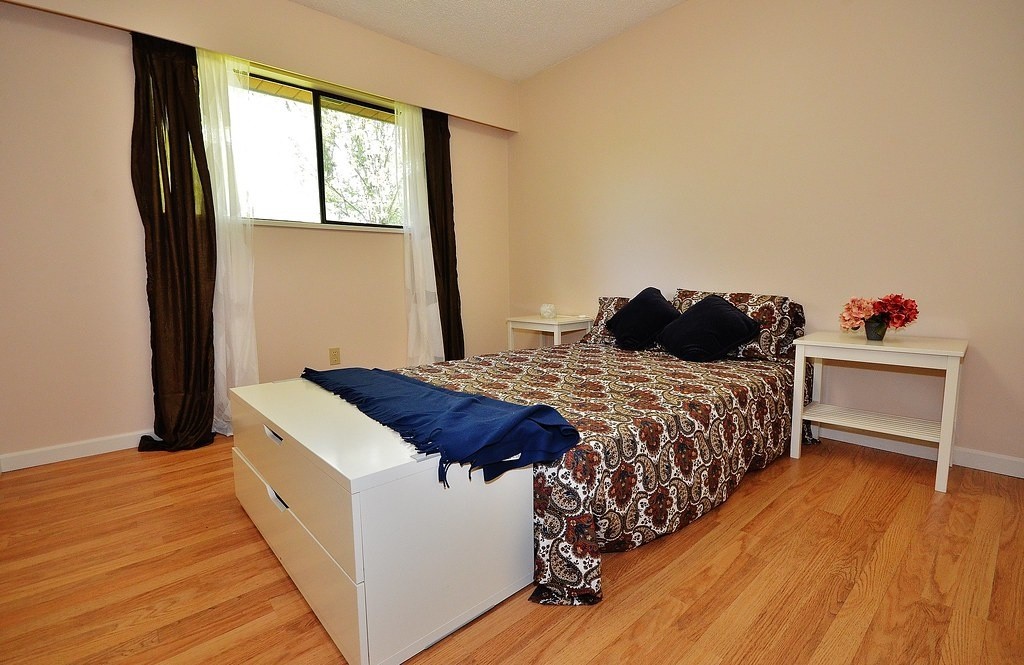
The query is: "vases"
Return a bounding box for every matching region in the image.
[864,319,888,341]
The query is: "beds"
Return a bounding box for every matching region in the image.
[384,287,821,605]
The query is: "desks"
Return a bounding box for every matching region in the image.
[790,328,969,494]
[505,313,593,351]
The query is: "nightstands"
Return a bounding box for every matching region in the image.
[227,376,535,665]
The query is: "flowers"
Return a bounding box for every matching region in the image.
[838,293,919,331]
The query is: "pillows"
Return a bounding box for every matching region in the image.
[656,294,760,362]
[577,296,632,344]
[669,289,794,361]
[606,287,681,351]
